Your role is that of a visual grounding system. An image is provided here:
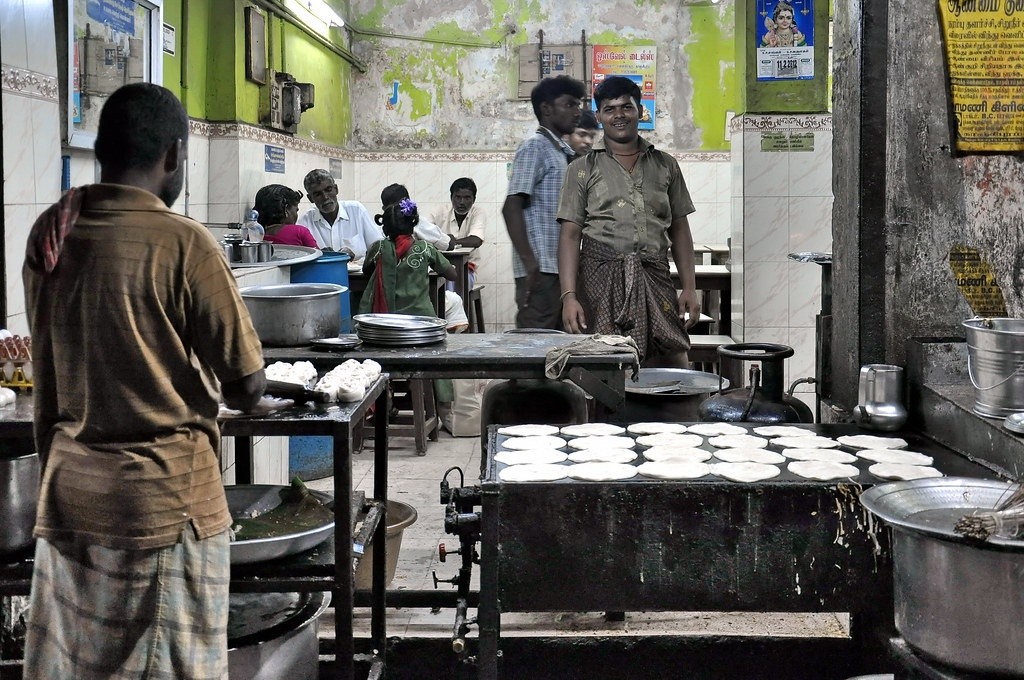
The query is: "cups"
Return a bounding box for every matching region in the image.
[217,233,272,264]
[703,253,712,265]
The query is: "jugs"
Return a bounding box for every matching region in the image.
[854,364,909,431]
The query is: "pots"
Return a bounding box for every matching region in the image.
[847,472,1024,677]
[624,367,730,422]
[237,282,348,347]
[0,436,40,551]
[225,590,332,680]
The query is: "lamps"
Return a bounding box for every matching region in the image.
[297,0,345,29]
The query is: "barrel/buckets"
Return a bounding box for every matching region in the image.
[289,251,351,481]
[961,317,1024,421]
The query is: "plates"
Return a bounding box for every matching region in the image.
[309,311,448,350]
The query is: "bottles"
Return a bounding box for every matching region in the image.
[240,209,265,242]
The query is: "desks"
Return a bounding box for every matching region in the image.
[223,225,729,621]
[0,371,395,680]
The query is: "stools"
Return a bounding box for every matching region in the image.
[687,334,738,373]
[685,313,717,372]
[353,379,439,457]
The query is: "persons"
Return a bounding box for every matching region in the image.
[555,75,701,369]
[254,184,355,262]
[358,197,457,318]
[296,169,384,260]
[21,82,267,680]
[378,184,469,334]
[563,110,598,165]
[503,76,586,331]
[428,178,485,293]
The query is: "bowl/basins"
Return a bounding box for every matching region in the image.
[302,499,417,592]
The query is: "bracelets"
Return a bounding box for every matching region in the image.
[560,290,576,303]
